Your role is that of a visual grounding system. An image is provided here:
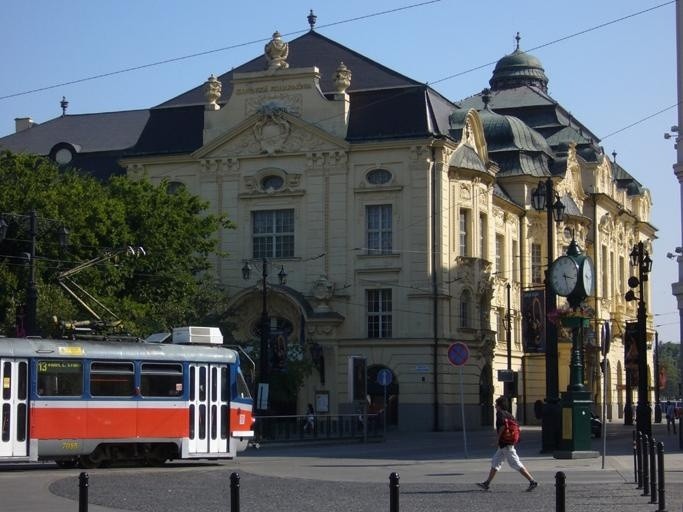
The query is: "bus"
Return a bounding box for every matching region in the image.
[0,325,256,467]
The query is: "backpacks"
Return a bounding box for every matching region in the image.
[502,416,521,444]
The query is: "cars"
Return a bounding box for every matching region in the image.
[671,401,683,418]
[590,411,602,437]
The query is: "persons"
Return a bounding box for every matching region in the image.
[474,397,538,492]
[631,398,682,434]
[303,403,314,431]
[360,393,383,443]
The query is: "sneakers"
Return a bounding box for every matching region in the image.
[526,481,538,492]
[476,482,489,490]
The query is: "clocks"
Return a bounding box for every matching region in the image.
[582,255,594,297]
[549,255,579,295]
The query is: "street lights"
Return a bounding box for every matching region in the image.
[0,210,69,336]
[532,175,565,452]
[628,240,652,439]
[242,256,287,415]
